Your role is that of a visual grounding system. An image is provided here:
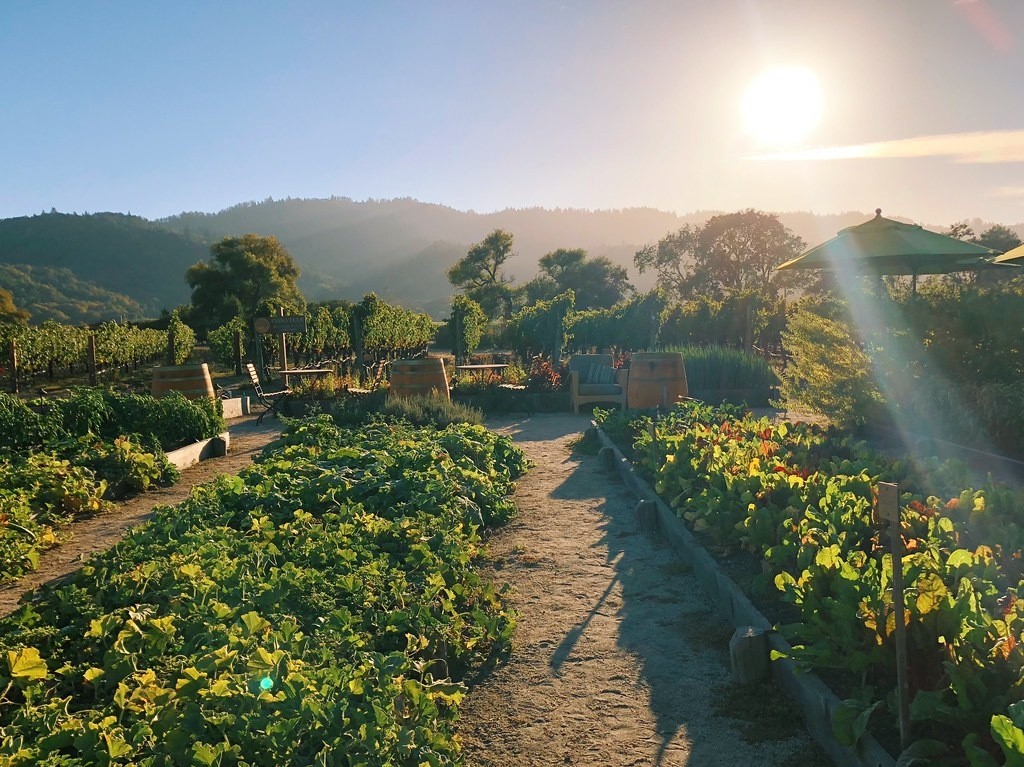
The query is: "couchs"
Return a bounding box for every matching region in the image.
[566,354,628,415]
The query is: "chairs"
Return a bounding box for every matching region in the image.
[344,361,386,423]
[497,356,539,417]
[246,363,294,426]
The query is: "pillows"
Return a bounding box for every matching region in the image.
[587,363,617,384]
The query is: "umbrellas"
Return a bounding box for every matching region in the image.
[775,207,1024,348]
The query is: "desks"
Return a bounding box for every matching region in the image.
[278,370,335,417]
[456,364,509,399]
